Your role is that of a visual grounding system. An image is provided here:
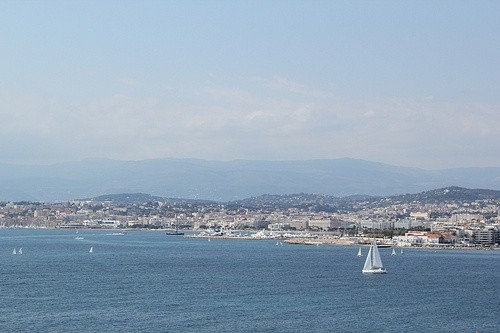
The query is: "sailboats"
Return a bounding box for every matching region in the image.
[361,239,387,273]
[89,247,93,253]
[391,248,397,256]
[12,247,23,255]
[400,248,404,255]
[356,248,364,258]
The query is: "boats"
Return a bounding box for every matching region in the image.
[74,236,84,240]
[166,231,184,235]
[111,231,127,236]
